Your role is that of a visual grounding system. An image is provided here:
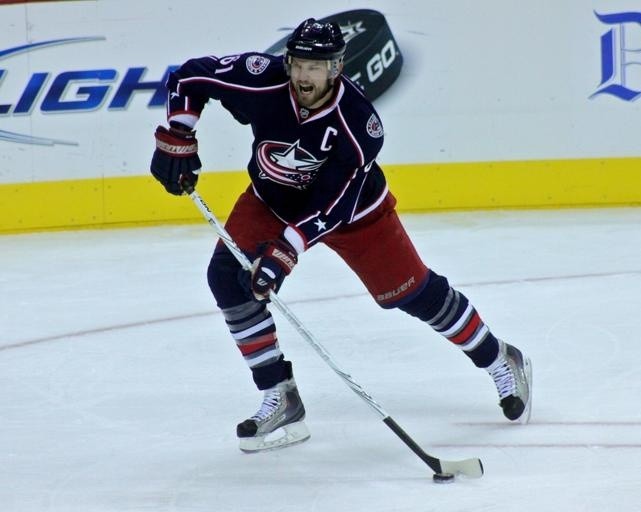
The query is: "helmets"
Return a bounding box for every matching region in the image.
[285,18,346,61]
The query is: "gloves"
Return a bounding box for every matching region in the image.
[237,241,301,304]
[150,125,202,197]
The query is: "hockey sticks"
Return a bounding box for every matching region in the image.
[179,176,485,477]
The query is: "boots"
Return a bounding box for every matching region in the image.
[236,361,306,437]
[462,334,528,421]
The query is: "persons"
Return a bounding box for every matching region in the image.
[150,17,530,438]
